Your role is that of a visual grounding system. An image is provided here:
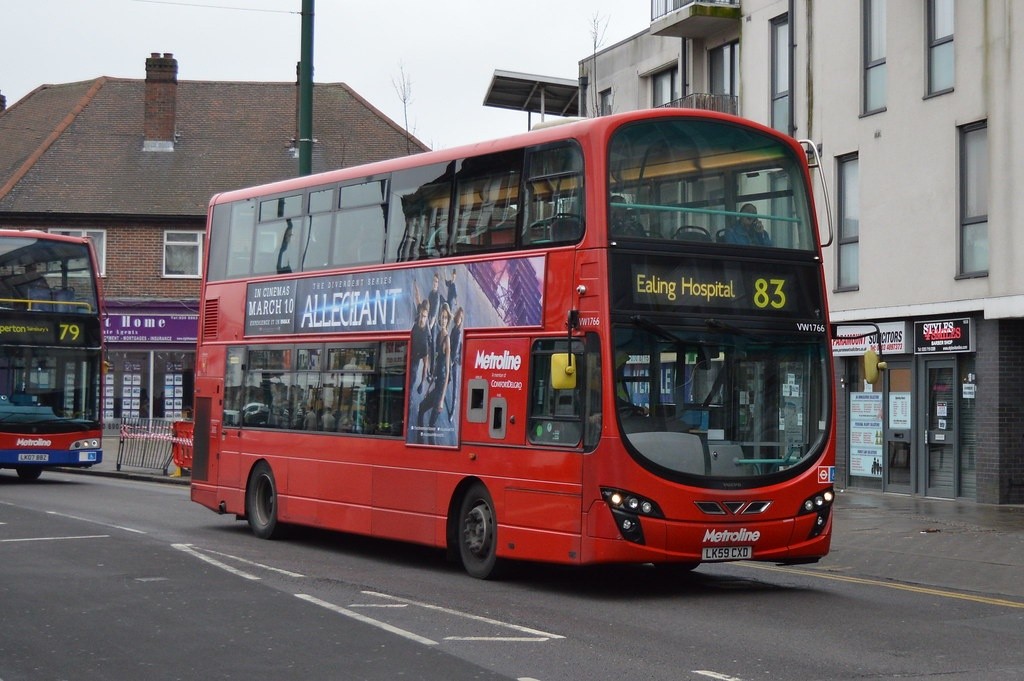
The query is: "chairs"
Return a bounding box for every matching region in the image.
[28,285,77,313]
[357,213,769,263]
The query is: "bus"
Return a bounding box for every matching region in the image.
[187,107,889,581]
[0,227,112,481]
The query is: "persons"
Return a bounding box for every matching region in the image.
[303,407,336,431]
[727,204,772,246]
[410,267,463,445]
[577,369,650,433]
